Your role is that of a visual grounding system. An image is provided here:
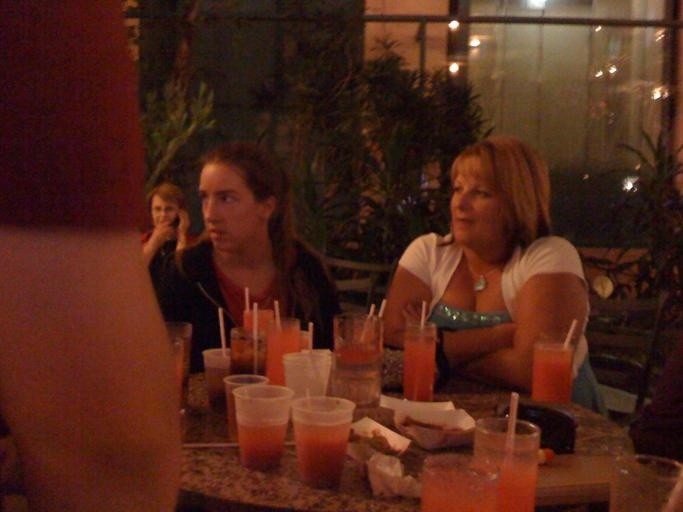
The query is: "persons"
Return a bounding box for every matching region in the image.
[0,3,189,511]
[154,139,341,376]
[379,135,608,414]
[139,181,200,271]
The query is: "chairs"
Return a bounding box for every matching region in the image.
[578,289,672,450]
[316,256,397,334]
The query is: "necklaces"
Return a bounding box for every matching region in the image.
[468,264,496,292]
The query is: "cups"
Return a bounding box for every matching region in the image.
[401,320,437,403]
[417,416,542,512]
[199,307,356,491]
[162,320,194,394]
[329,312,383,412]
[530,329,576,412]
[607,455,682,512]
